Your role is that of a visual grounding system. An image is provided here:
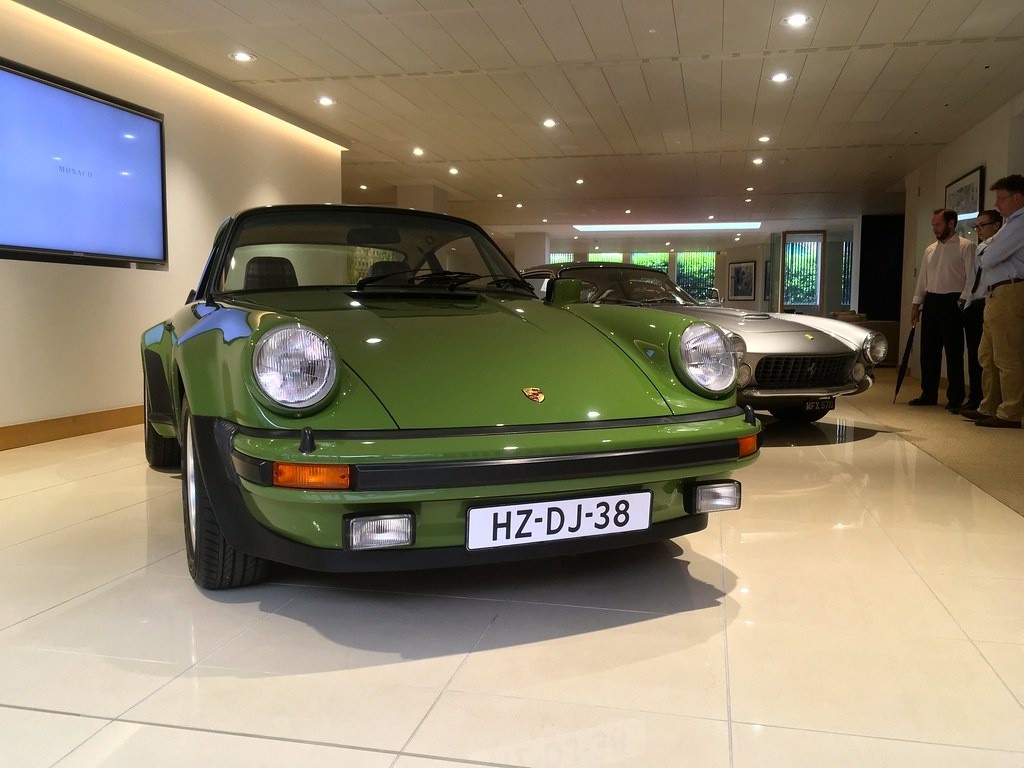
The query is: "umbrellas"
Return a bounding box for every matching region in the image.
[892,307,925,404]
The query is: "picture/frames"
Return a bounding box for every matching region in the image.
[944,165,984,246]
[764,260,771,301]
[728,261,756,301]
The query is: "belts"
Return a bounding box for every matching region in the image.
[987,278,1024,292]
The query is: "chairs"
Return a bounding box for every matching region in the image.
[242,256,298,292]
[366,261,415,287]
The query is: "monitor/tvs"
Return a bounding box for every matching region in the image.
[0,56,170,271]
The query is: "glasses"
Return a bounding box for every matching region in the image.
[996,193,1016,201]
[972,220,999,231]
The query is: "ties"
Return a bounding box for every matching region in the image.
[971,246,986,295]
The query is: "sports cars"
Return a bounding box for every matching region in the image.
[502,262,889,423]
[142,204,762,589]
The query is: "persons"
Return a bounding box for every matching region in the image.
[950,209,1004,415]
[907,209,978,410]
[961,174,1023,428]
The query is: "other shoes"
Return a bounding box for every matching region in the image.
[908,398,937,406]
[945,402,962,410]
[959,409,994,420]
[949,403,971,414]
[974,416,1021,428]
